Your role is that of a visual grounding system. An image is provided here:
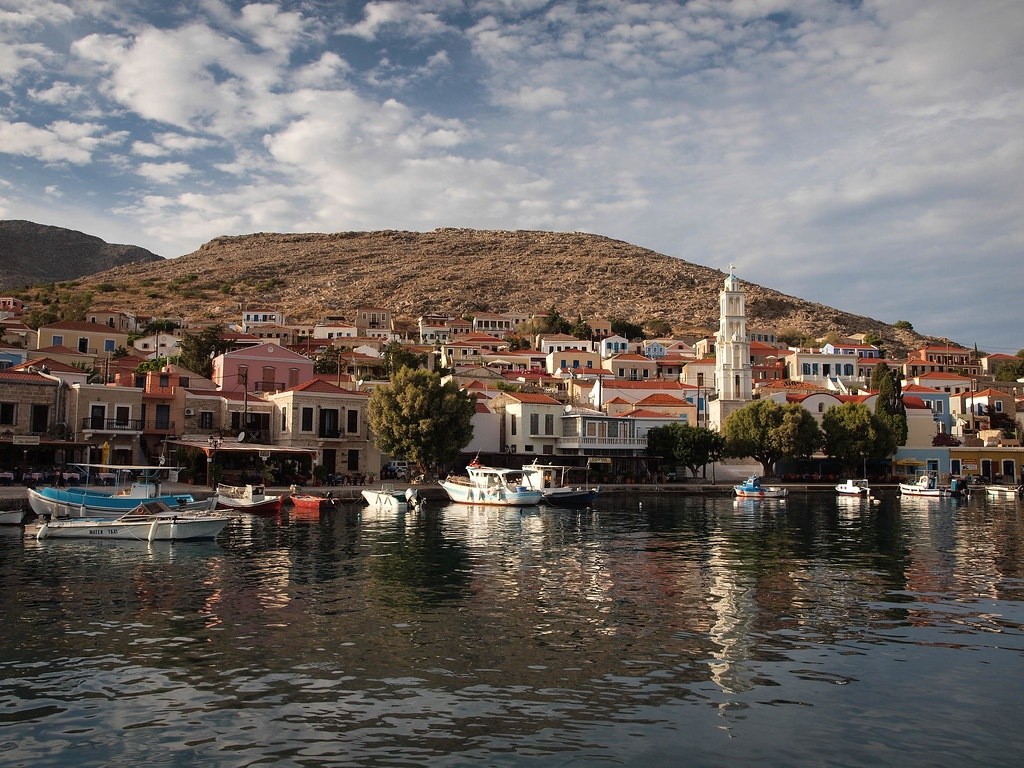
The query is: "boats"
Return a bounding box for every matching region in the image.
[359,483,427,513]
[834,478,871,496]
[288,485,339,509]
[984,482,1024,498]
[521,457,599,506]
[27,463,195,518]
[898,475,952,497]
[215,483,282,513]
[25,499,232,541]
[942,478,976,497]
[437,446,542,506]
[0,510,25,524]
[732,472,789,498]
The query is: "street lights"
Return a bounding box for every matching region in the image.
[207,430,224,492]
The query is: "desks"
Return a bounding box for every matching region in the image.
[61,472,79,486]
[0,473,14,485]
[23,471,43,486]
[94,473,116,486]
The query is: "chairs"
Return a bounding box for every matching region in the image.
[321,474,366,486]
